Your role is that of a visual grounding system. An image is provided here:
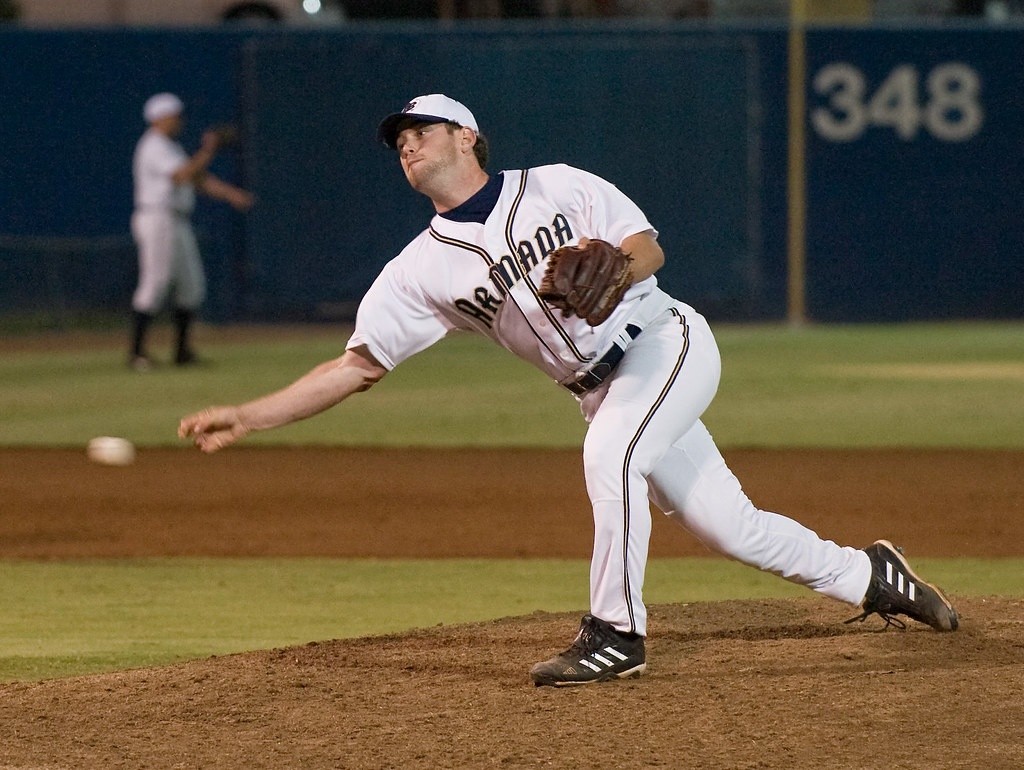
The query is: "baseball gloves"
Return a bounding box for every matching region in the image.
[536,238,634,327]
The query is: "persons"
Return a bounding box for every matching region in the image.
[177,95,957,685]
[129,93,252,368]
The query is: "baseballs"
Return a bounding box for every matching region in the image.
[88,436,136,466]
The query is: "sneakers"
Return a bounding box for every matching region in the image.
[843,540,958,633]
[530,614,646,687]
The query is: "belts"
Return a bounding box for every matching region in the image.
[563,323,641,395]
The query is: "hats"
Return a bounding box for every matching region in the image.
[376,94,479,151]
[143,94,183,122]
